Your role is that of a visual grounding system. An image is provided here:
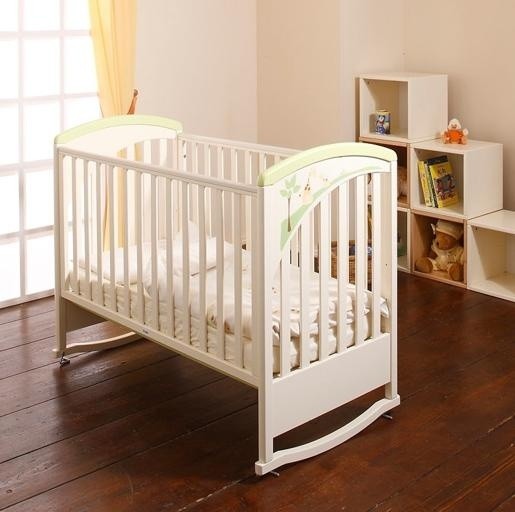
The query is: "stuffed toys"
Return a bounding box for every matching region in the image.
[441,118,469,145]
[415,220,464,282]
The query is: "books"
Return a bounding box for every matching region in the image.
[418,154,459,208]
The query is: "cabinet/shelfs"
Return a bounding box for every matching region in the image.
[356,72,515,303]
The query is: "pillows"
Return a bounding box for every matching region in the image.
[71,221,234,285]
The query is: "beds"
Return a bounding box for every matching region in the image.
[53,112,401,478]
